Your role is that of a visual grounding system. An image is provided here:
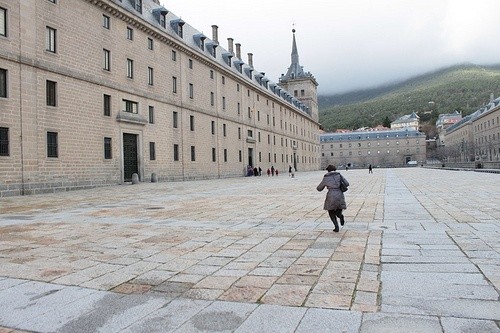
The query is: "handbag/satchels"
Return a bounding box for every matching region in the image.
[340,174,348,192]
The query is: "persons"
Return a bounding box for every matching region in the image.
[289,165,295,177]
[247,165,262,176]
[316,164,349,232]
[346,165,348,171]
[267,165,278,176]
[369,163,373,174]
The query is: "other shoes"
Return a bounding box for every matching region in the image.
[340,215,344,226]
[333,226,339,231]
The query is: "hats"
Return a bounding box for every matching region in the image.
[326,164,337,170]
